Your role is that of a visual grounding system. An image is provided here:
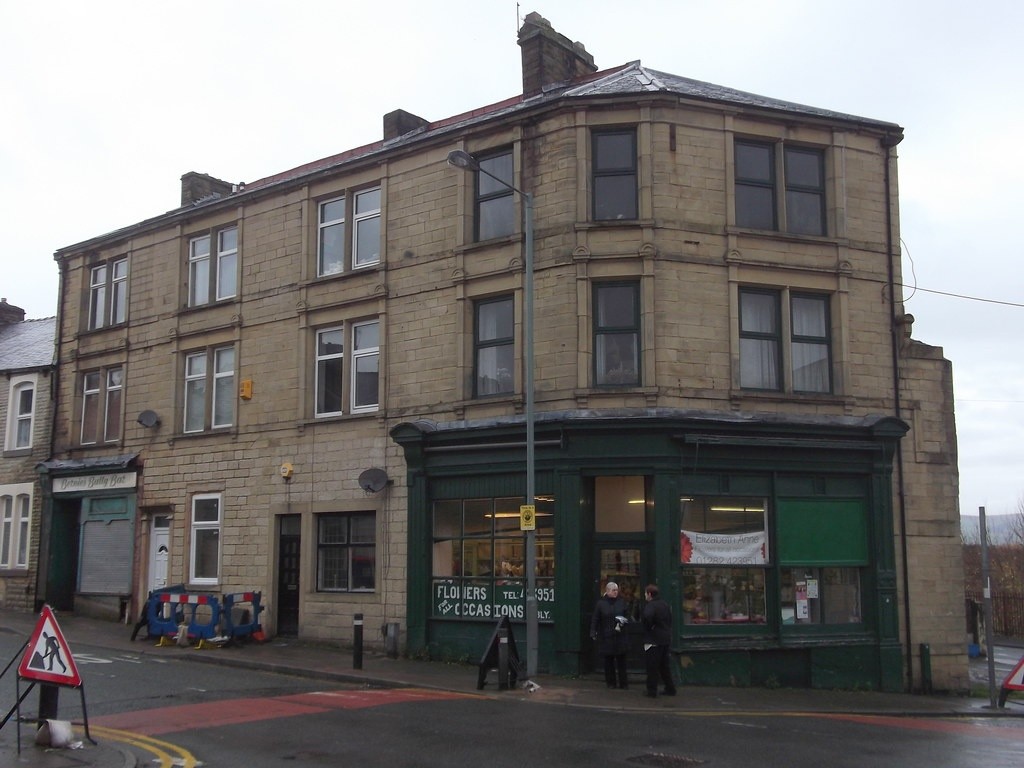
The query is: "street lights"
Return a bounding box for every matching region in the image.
[447,148,539,693]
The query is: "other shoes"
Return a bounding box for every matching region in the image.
[608,684,617,690]
[659,689,676,697]
[617,684,628,690]
[644,690,656,698]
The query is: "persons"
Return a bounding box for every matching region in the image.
[588,583,633,690]
[640,584,677,698]
